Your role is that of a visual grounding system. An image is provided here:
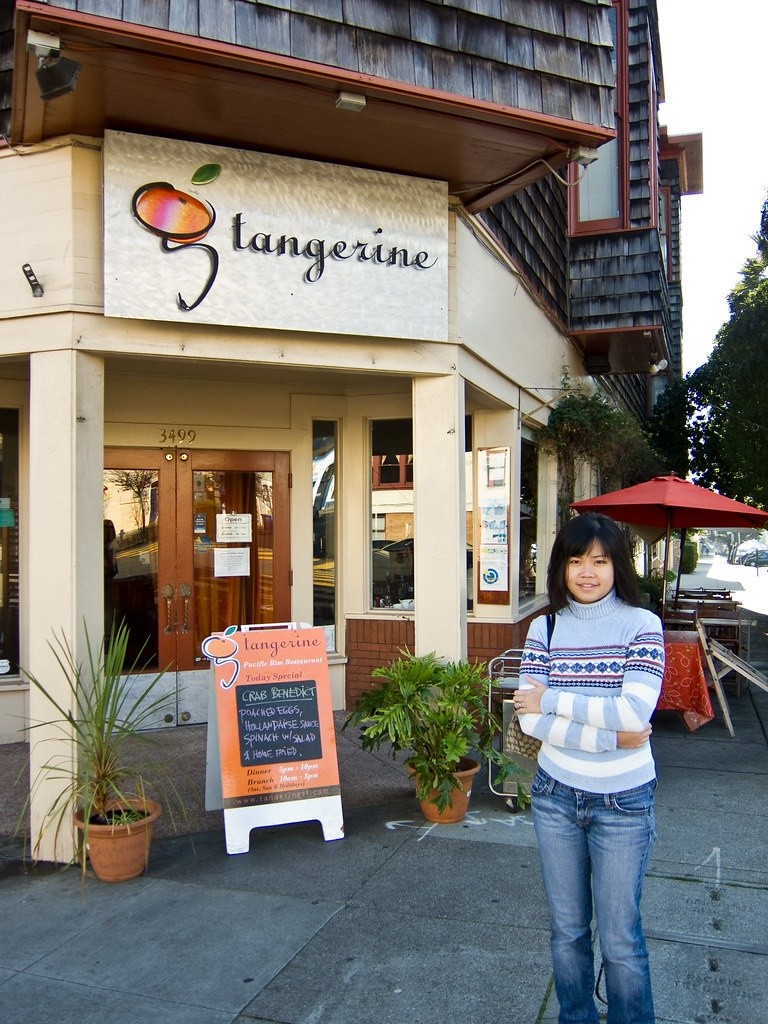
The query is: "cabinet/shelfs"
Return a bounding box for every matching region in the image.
[488,648,539,813]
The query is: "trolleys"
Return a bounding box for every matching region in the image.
[487,649,535,811]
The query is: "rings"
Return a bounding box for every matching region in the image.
[519,702,522,708]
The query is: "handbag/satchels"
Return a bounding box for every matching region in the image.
[506,614,556,760]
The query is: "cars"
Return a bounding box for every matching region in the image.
[736,548,768,567]
[372,537,473,606]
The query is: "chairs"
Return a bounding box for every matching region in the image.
[660,587,741,699]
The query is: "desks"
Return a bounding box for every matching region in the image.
[655,631,715,734]
[660,587,758,688]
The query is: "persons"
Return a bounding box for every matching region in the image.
[513,511,664,1024]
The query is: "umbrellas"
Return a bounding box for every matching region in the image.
[567,471,768,631]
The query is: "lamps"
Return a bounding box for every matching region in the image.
[25,29,83,102]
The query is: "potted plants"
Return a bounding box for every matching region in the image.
[12,607,197,884]
[341,644,534,823]
